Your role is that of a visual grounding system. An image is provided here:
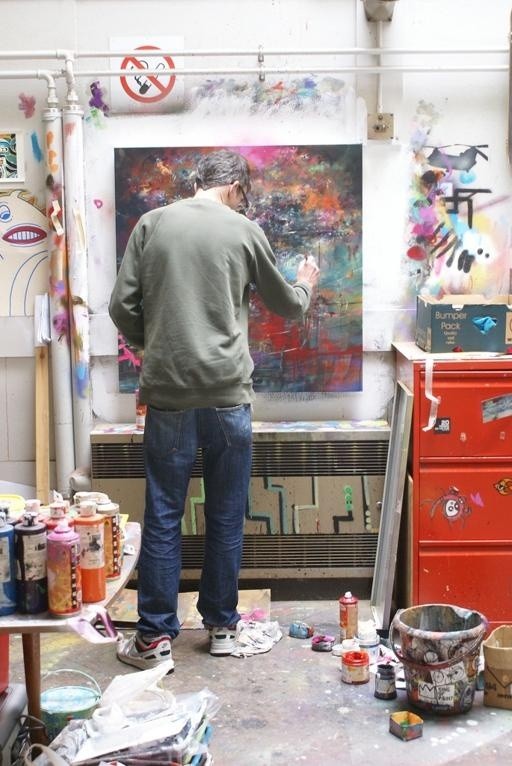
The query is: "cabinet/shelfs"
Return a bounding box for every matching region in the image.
[392,348,512,641]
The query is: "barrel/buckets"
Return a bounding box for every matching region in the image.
[386,600,489,718]
[38,668,101,744]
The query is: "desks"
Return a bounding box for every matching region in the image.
[0,520,143,765]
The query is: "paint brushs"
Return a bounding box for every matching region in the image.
[305,255,307,261]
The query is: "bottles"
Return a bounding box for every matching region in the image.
[338,591,360,643]
[373,664,397,700]
[135,389,147,431]
[339,649,371,685]
[0,490,125,619]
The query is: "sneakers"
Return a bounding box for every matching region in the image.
[209,619,246,656]
[117,631,174,675]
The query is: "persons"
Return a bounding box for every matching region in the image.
[108,148,322,678]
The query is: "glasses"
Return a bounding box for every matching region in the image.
[230,182,250,208]
